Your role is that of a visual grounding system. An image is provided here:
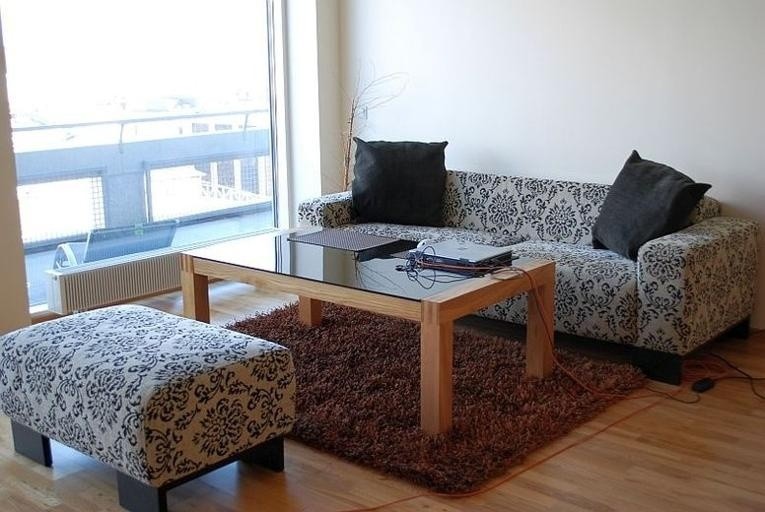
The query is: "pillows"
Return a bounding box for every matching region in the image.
[591,149,713,261]
[348,136,447,228]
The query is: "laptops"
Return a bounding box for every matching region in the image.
[410,239,512,268]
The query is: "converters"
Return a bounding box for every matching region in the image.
[690,378,714,392]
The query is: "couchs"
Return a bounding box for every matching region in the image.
[295,163,759,386]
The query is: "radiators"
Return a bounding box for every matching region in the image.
[44,246,184,315]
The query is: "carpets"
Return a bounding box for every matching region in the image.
[214,298,647,494]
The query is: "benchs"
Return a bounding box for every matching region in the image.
[1,303,296,512]
[50,226,176,268]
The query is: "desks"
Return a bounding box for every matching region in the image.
[176,226,559,434]
